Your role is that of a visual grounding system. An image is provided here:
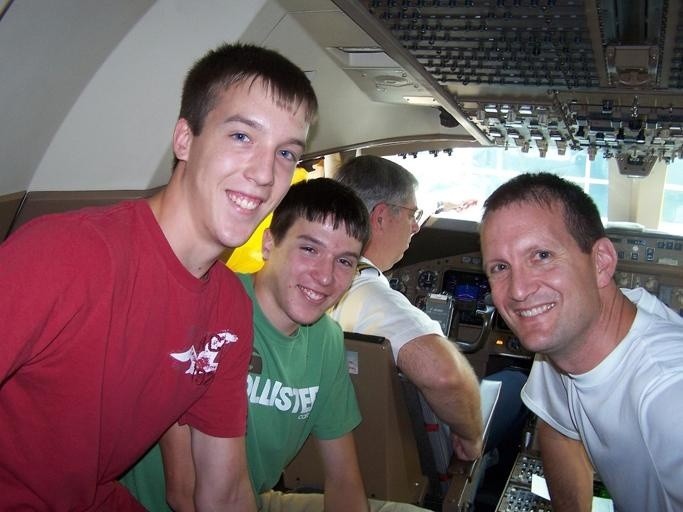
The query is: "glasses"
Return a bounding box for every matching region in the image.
[385,202,423,225]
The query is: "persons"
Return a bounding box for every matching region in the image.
[0,39,321,512]
[324,154,486,462]
[477,170,683,512]
[113,176,371,512]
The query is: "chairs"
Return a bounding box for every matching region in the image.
[281,331,501,512]
